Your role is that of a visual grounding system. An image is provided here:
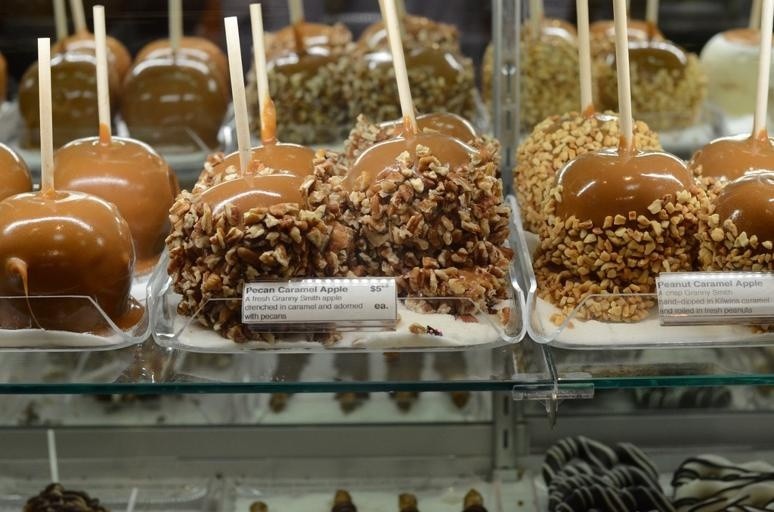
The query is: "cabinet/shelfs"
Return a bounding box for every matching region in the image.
[0,152,772,512]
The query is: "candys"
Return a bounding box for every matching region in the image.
[0,0,774,512]
